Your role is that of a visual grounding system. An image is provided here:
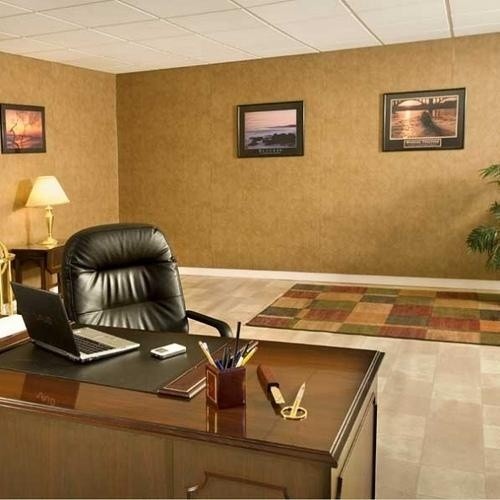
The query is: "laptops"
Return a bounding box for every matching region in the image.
[10,281,141,363]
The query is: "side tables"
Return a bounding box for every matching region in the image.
[10,245,64,314]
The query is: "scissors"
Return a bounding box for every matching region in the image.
[280,382,308,420]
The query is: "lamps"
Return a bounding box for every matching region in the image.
[25,175,69,245]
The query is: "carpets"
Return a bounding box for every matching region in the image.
[246,283,500,346]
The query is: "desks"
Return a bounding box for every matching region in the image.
[0,313,384,499]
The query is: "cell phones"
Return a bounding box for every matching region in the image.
[150,343,186,359]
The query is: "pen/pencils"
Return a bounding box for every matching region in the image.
[198,321,259,371]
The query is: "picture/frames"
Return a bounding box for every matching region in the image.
[383,87,466,151]
[237,100,304,156]
[1,103,46,153]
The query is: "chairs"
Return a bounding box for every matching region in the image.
[63,223,232,338]
